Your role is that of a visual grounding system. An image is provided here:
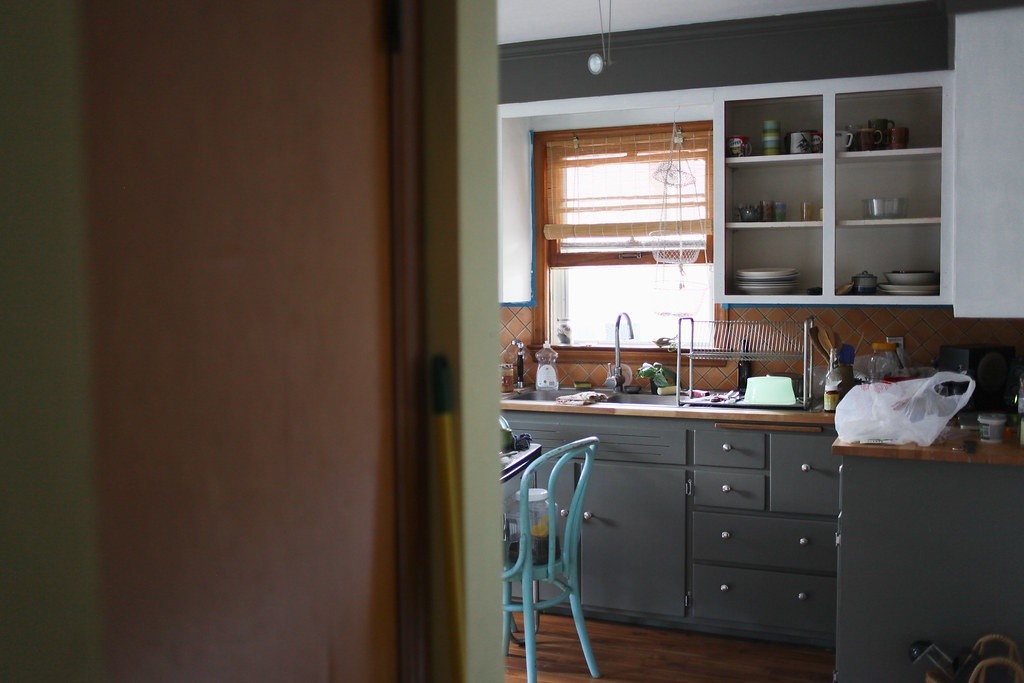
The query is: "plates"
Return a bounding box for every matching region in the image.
[735,266,800,294]
[876,270,939,294]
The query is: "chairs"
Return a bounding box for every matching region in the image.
[501,436,603,683]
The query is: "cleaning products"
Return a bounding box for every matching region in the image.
[535,341,559,391]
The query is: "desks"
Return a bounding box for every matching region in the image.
[498,441,544,502]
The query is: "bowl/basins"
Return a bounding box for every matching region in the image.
[860,195,910,221]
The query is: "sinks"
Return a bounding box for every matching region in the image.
[598,392,690,409]
[502,386,613,404]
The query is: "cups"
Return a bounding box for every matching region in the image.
[738,198,812,221]
[729,114,910,156]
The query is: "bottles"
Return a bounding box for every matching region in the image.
[736,339,752,394]
[507,488,560,566]
[556,317,571,344]
[867,341,900,387]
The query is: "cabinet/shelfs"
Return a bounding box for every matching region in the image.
[499,410,694,631]
[676,316,816,410]
[690,425,842,652]
[710,70,954,305]
[829,456,1024,683]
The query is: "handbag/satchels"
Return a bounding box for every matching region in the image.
[832,371,977,449]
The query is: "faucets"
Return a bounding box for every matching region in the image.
[509,339,526,391]
[605,312,636,393]
